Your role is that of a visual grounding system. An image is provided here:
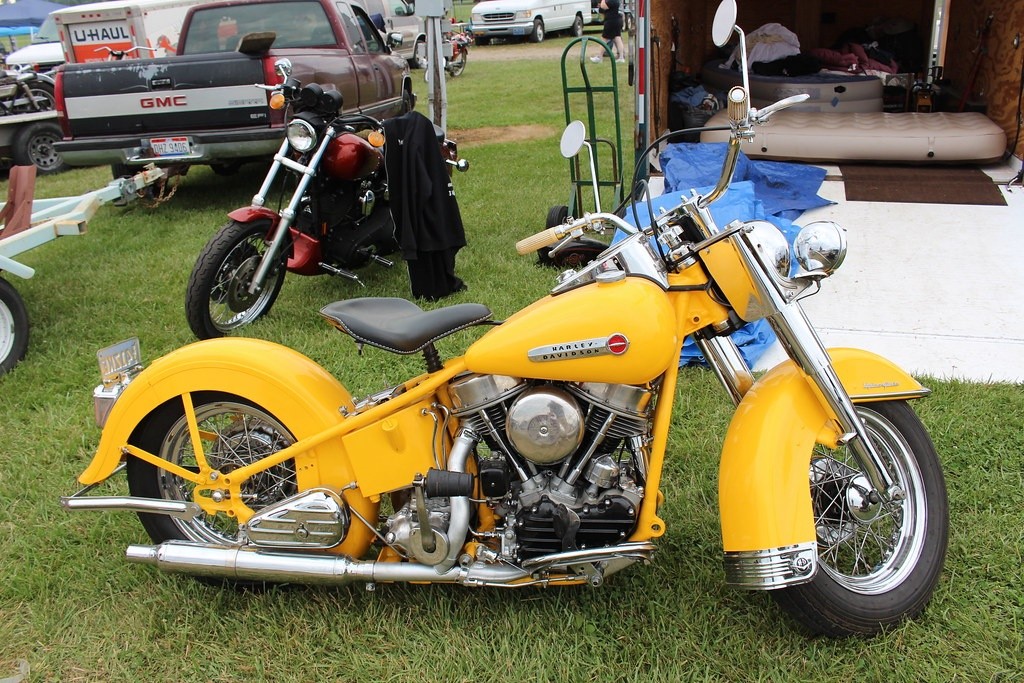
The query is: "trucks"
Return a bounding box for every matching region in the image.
[628,0,1024,389]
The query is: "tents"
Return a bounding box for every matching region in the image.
[0,0,70,44]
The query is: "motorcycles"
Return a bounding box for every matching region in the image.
[424,31,472,84]
[186,54,472,338]
[73,1,954,641]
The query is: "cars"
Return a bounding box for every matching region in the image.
[0,5,81,178]
[589,1,633,31]
[354,1,428,69]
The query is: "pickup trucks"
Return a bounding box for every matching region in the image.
[50,0,417,201]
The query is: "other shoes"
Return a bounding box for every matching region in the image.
[614,57,626,63]
[590,56,603,64]
[602,52,611,57]
[614,54,625,58]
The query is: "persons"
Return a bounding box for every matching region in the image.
[589,0,625,64]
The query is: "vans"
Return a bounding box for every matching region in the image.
[469,1,593,46]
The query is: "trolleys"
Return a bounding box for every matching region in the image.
[545,37,627,266]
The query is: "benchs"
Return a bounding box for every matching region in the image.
[696,103,1006,171]
